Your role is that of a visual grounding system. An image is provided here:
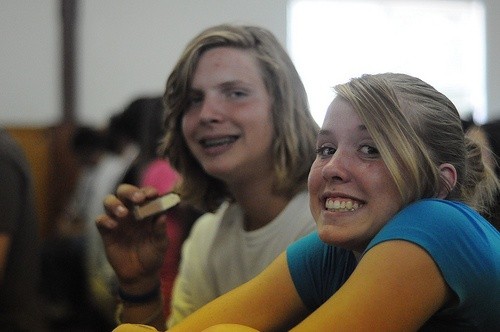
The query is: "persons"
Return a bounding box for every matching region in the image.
[111,72,500,331]
[0,95,188,332]
[93,23,322,332]
[459,116,499,233]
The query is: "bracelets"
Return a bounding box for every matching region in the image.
[108,279,161,303]
